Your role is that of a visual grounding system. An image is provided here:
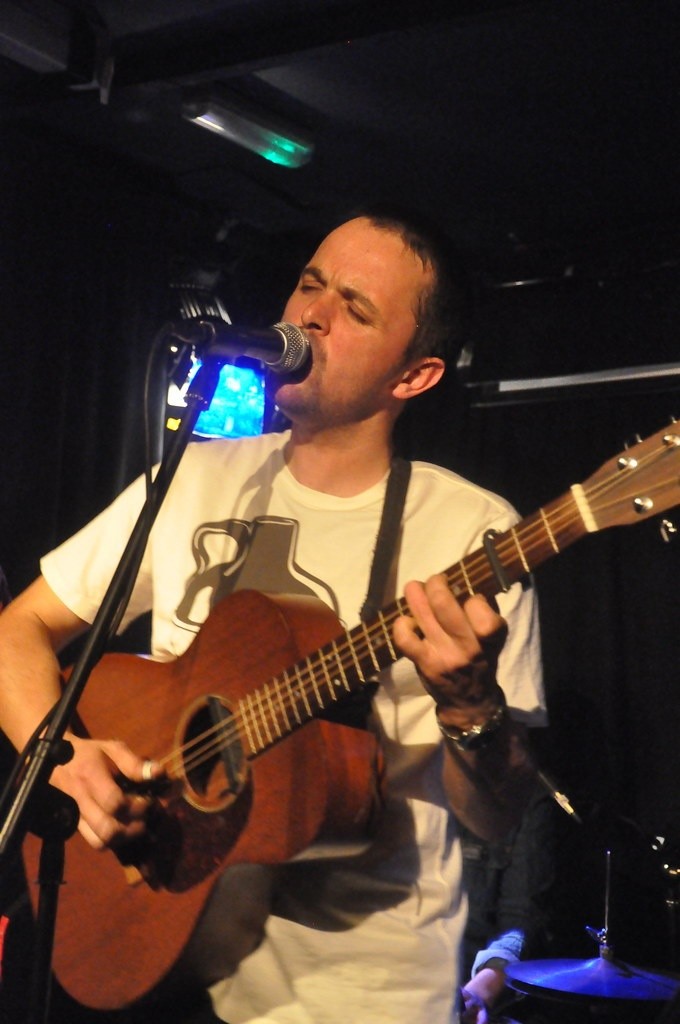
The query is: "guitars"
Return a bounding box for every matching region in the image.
[17,417,679,1021]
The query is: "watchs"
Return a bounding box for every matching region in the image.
[435,686,507,751]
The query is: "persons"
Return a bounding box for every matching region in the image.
[0,201,551,1024]
[457,770,553,1024]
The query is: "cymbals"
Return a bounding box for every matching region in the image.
[500,941,678,1018]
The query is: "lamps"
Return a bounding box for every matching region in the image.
[180,81,320,173]
[0,0,114,91]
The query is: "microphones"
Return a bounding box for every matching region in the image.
[172,321,316,380]
